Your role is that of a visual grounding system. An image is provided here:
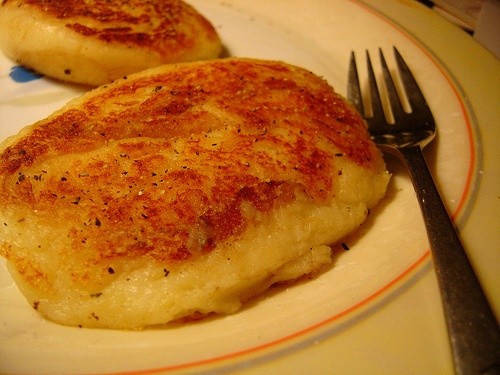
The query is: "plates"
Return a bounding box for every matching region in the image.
[0,0,485,375]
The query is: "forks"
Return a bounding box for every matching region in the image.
[346,44,500,375]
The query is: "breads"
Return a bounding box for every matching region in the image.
[0,57,393,331]
[0,0,224,88]
